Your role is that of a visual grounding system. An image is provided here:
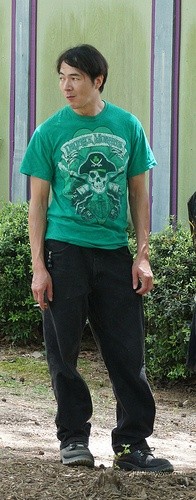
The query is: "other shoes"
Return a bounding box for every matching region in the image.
[112,445,175,473]
[60,441,94,467]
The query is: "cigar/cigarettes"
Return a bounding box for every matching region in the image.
[34,303,47,307]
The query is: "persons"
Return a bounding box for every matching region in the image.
[19,44,174,472]
